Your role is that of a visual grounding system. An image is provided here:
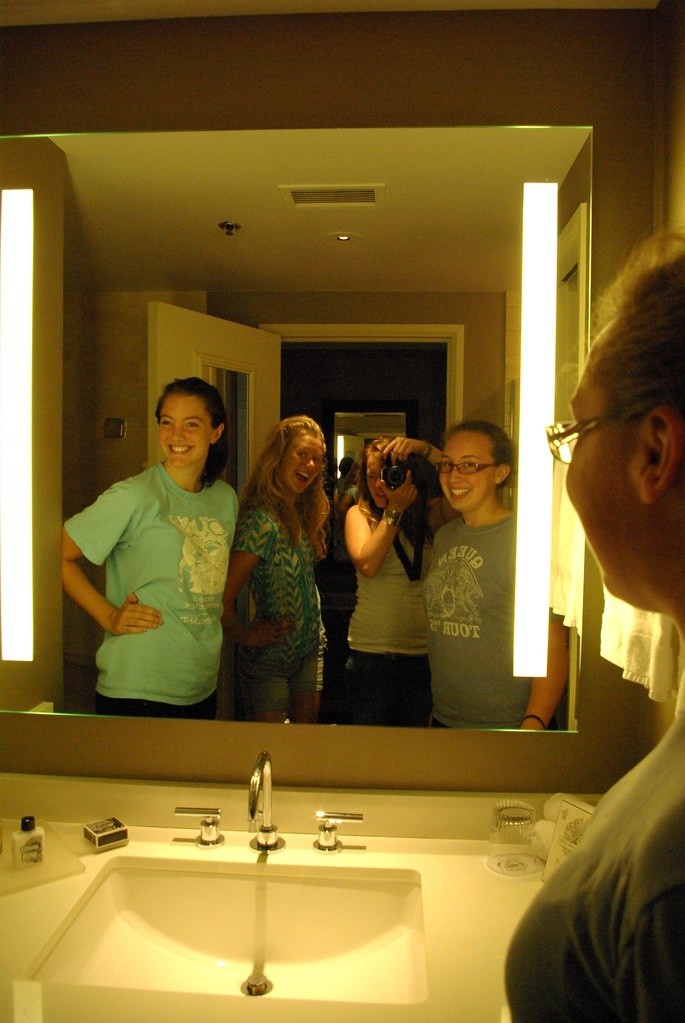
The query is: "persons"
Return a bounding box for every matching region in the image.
[505,229,685,1023]
[220,414,331,724]
[343,435,463,727]
[334,457,360,546]
[61,376,240,720]
[422,420,569,731]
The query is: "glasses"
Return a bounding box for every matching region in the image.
[546,416,621,465]
[435,461,497,474]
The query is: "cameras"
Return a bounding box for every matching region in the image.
[380,452,411,490]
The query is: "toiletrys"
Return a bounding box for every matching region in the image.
[10,815,46,871]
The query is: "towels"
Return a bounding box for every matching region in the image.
[598,578,685,719]
[549,453,586,637]
[529,792,597,862]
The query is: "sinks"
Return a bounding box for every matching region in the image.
[24,854,430,1006]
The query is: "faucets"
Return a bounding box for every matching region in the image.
[274,713,291,723]
[245,749,287,855]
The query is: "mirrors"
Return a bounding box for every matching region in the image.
[0,124,596,796]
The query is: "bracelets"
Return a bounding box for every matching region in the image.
[423,441,432,458]
[381,509,401,528]
[520,715,548,730]
